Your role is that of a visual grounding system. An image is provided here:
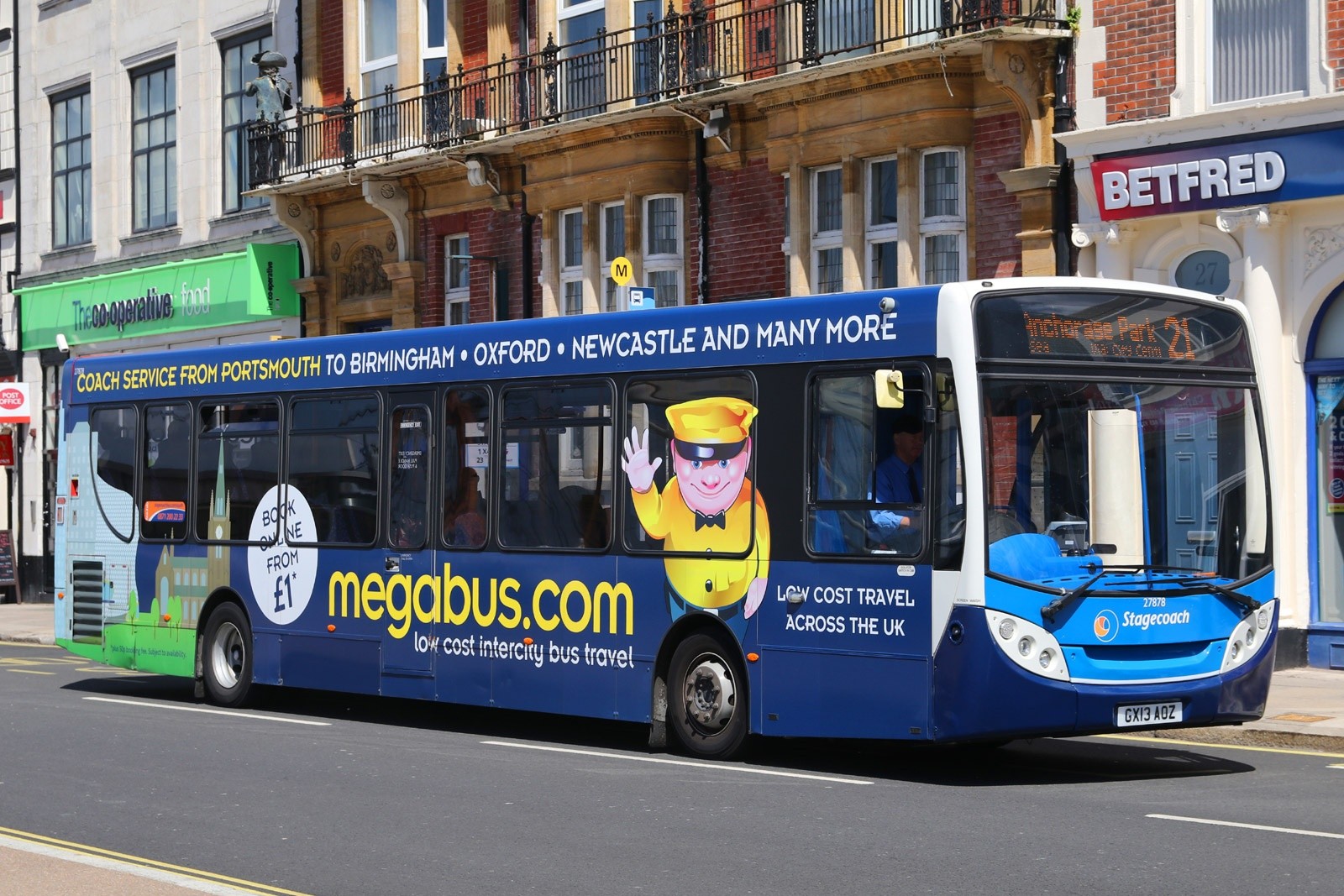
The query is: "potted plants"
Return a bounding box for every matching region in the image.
[696,37,724,90]
[463,97,497,140]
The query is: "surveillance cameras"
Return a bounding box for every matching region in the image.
[56,334,68,353]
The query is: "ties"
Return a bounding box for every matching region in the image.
[907,468,922,516]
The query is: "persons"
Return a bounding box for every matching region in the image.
[442,467,488,544]
[867,416,951,554]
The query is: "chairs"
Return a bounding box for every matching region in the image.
[97,439,606,547]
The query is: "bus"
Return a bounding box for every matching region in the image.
[54,275,1279,761]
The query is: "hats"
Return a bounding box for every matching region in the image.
[891,415,923,434]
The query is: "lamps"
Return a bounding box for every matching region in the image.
[703,102,732,139]
[465,154,490,186]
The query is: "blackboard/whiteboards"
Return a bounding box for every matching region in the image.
[0,529,20,585]
